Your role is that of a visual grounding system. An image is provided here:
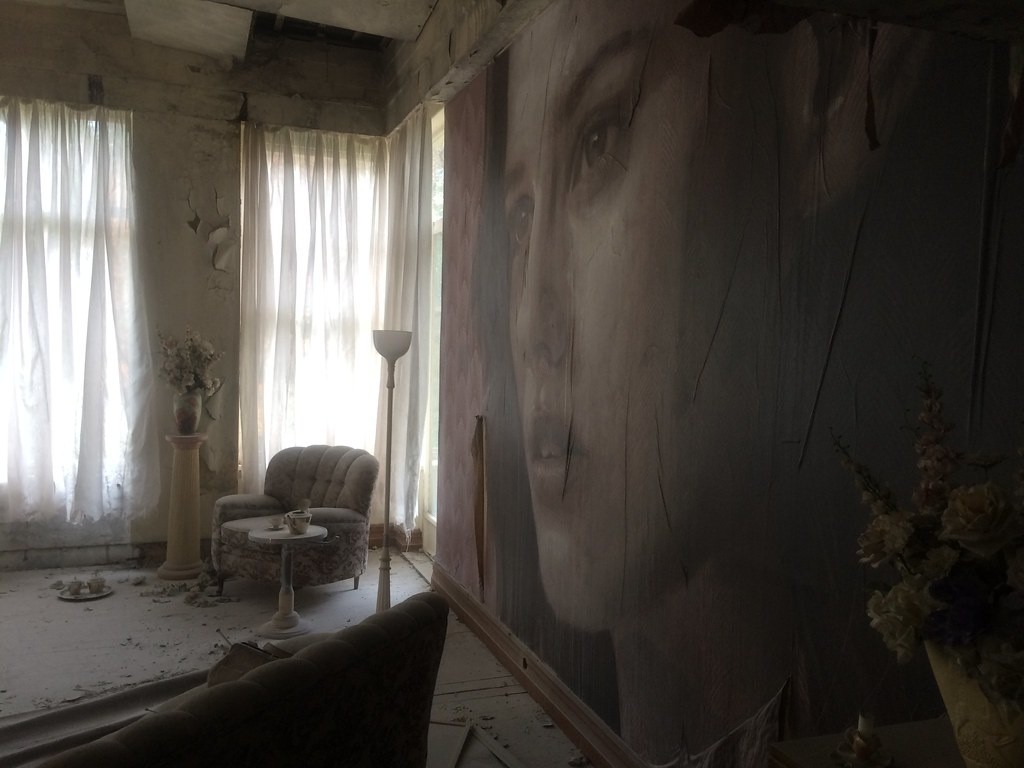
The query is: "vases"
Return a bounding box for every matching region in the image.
[923,632,1024,768]
[172,392,204,429]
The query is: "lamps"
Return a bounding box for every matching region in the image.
[373,329,413,608]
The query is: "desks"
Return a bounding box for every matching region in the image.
[764,714,1024,768]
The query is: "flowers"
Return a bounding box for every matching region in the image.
[824,357,1024,698]
[152,330,223,395]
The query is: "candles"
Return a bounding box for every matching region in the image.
[857,710,877,739]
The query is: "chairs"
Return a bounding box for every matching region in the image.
[211,445,378,600]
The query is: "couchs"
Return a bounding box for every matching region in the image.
[0,591,449,768]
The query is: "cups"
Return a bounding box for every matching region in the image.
[67,580,84,594]
[87,577,106,593]
[270,518,283,527]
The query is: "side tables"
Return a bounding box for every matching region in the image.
[247,526,329,638]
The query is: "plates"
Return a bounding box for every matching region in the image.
[268,526,284,531]
[58,585,113,599]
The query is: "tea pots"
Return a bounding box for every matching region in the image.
[285,510,312,535]
